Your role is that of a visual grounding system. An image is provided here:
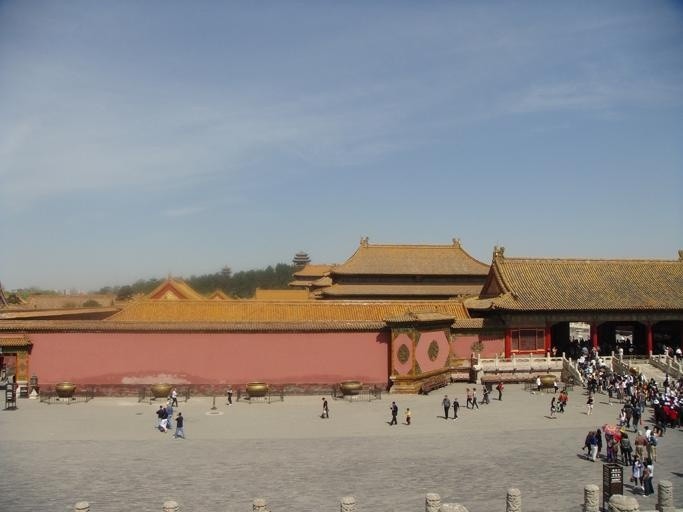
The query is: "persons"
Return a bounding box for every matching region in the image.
[156,388,184,438]
[405,407,411,424]
[535,336,683,497]
[322,398,329,418]
[227,384,233,405]
[442,382,504,419]
[390,402,398,425]
